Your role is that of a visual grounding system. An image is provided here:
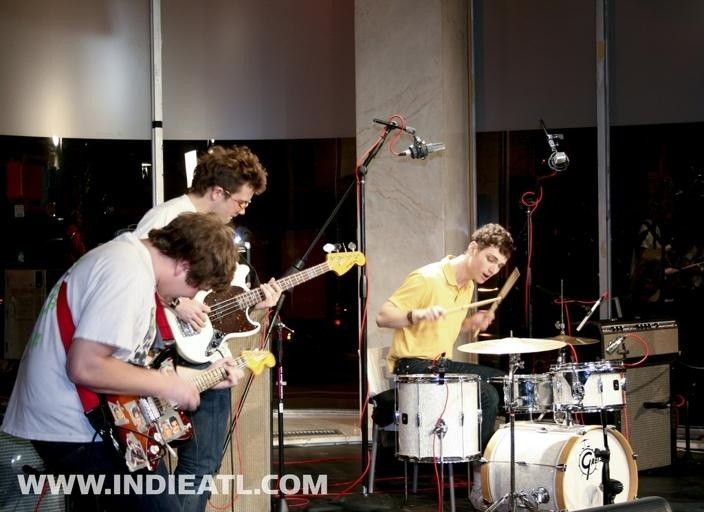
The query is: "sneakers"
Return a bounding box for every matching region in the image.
[471,490,484,509]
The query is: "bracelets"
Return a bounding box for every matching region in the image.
[406,309,416,325]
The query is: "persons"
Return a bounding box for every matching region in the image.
[373,220,522,489]
[118,147,283,512]
[129,406,147,433]
[111,406,129,426]
[0,211,244,510]
[159,419,172,438]
[127,439,145,467]
[626,201,685,311]
[168,415,181,433]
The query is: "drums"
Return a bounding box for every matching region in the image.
[549,361,626,414]
[488,374,553,414]
[397,373,482,465]
[480,420,639,512]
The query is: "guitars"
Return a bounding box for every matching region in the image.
[101,348,277,473]
[163,241,366,365]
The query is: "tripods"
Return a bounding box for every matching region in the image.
[484,352,541,512]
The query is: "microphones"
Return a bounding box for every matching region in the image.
[406,140,447,159]
[546,150,572,174]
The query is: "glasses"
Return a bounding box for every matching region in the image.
[226,191,251,207]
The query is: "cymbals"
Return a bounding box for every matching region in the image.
[458,334,600,355]
